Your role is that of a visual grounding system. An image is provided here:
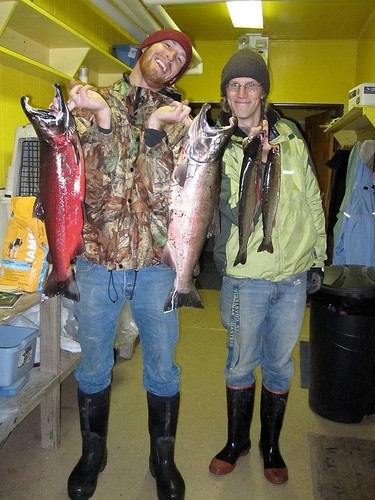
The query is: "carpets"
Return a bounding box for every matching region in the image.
[307,432,375,500]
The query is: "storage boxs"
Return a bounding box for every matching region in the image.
[0,324,40,387]
[348,83,375,112]
[112,44,141,69]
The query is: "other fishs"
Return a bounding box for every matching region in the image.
[159,105,238,314]
[257,144,282,256]
[18,82,88,302]
[233,136,265,266]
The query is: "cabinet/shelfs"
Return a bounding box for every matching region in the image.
[0,0,141,91]
[0,291,82,452]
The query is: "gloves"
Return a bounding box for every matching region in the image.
[307,267,324,294]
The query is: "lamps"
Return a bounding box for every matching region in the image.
[238,37,268,65]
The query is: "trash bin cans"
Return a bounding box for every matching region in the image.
[309,264,375,423]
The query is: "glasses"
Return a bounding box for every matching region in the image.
[225,82,263,92]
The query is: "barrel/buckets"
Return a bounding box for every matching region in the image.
[305,282,375,423]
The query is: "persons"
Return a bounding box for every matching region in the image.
[209,49,327,483]
[53,29,192,500]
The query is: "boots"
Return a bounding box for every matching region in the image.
[146,391,185,500]
[209,382,256,475]
[68,383,112,500]
[258,383,288,484]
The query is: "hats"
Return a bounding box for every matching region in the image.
[137,29,192,87]
[221,48,270,97]
